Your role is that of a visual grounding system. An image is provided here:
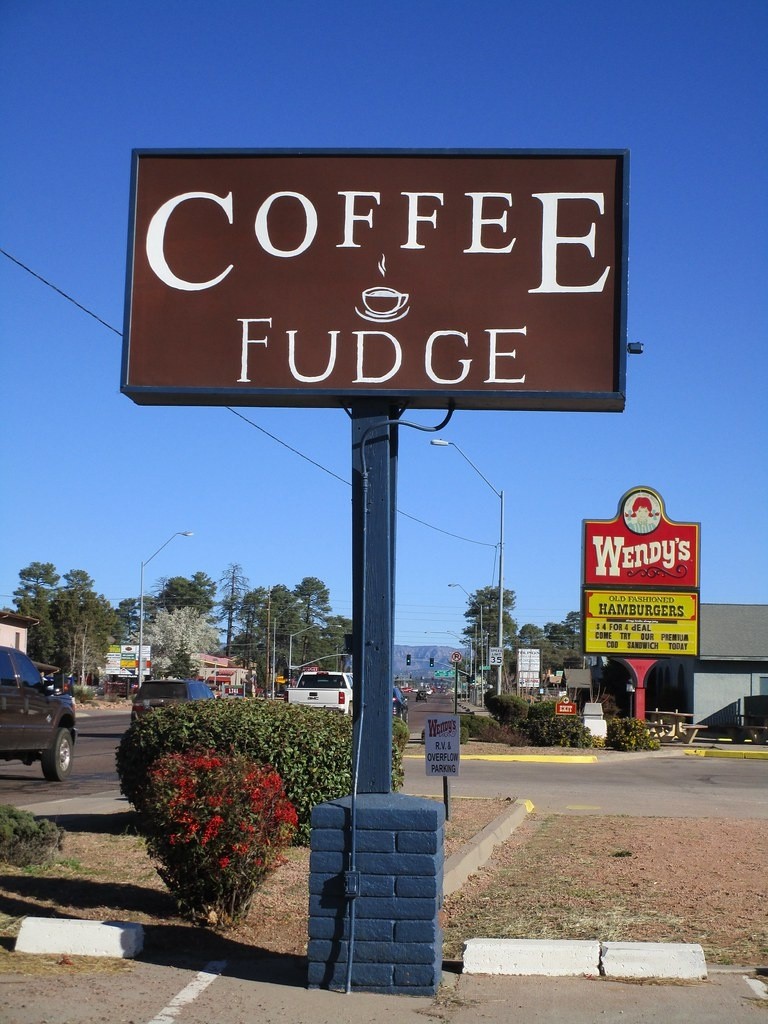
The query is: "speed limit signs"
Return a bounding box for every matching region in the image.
[489,647,504,665]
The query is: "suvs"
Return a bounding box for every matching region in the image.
[0,646,77,782]
[401,687,418,692]
[415,690,427,702]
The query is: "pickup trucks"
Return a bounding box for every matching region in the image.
[284,670,354,715]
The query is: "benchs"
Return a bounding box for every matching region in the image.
[729,725,767,744]
[645,721,708,744]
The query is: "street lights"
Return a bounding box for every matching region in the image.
[423,628,472,708]
[288,622,342,703]
[137,532,193,688]
[430,438,506,695]
[447,583,490,709]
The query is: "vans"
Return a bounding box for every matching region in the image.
[131,679,215,723]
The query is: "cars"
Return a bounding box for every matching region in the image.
[392,687,409,727]
[262,689,287,699]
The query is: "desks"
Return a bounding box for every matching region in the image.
[645,711,696,742]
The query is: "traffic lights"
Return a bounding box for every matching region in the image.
[429,657,435,668]
[406,654,412,665]
